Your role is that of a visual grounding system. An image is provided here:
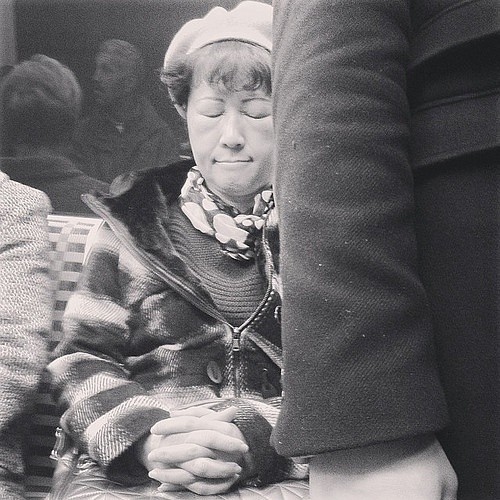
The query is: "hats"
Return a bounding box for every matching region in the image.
[162,0,274,120]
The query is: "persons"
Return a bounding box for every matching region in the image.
[75,38,179,185]
[0,170,55,500]
[270,1,500,500]
[46,1,311,500]
[0,53,111,215]
